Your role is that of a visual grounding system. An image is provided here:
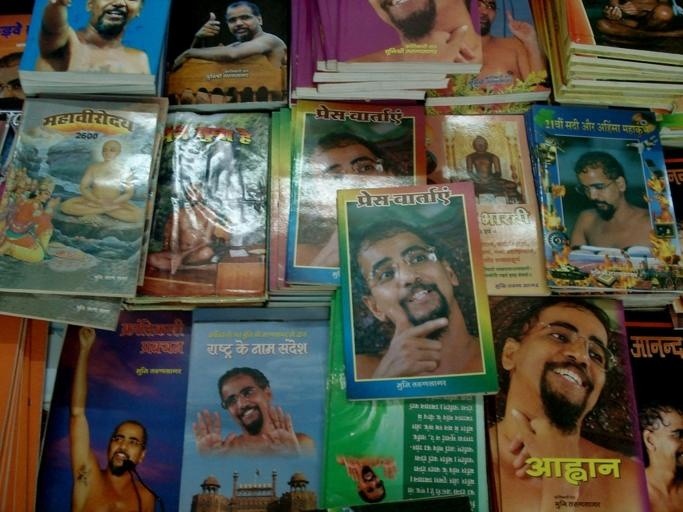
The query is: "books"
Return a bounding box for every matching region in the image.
[1,1,683,510]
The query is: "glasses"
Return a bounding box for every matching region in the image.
[575,185,611,194]
[536,322,616,372]
[112,437,144,446]
